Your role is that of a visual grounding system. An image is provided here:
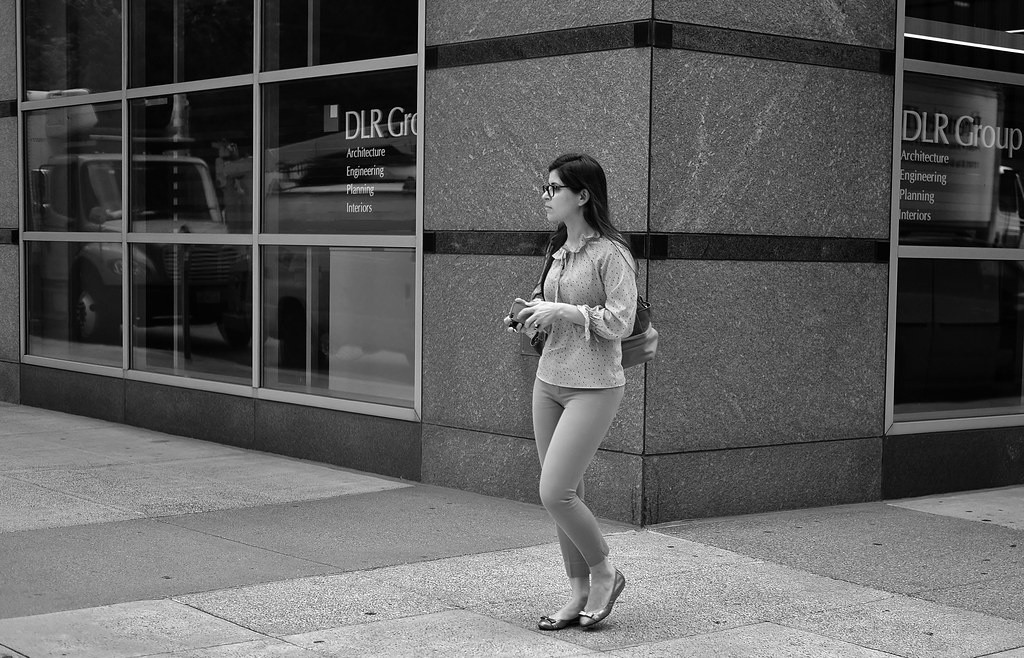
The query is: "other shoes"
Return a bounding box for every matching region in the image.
[538,614,581,631]
[579,568,625,626]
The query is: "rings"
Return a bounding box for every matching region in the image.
[534,322,538,327]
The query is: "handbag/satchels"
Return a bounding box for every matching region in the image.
[530,295,658,369]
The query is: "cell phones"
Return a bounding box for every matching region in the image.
[510,302,533,325]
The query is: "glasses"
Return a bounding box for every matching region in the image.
[542,185,567,198]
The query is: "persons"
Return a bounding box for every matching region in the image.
[502,151,660,632]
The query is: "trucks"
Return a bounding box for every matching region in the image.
[25,120,419,370]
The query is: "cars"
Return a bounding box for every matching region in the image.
[986,165,1024,253]
